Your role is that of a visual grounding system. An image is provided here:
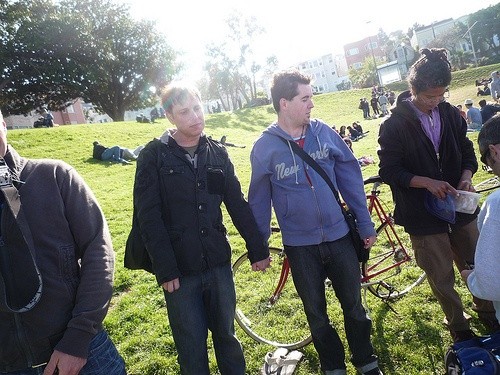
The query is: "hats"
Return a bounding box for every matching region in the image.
[465,99,473,105]
[477,112,500,154]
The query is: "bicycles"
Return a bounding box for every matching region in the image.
[230,175,426,351]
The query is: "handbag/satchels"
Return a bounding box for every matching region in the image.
[121,227,179,275]
[342,208,371,264]
[442,329,500,375]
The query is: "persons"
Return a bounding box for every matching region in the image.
[456,98,500,131]
[460,112,499,330]
[331,122,363,143]
[248,70,380,375]
[475,77,492,86]
[1,106,128,375]
[133,82,269,374]
[377,46,498,344]
[140,105,166,122]
[92,140,143,165]
[490,70,500,104]
[476,82,491,96]
[359,84,395,118]
[207,101,222,114]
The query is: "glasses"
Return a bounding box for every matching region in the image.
[480,143,497,165]
[418,89,451,106]
